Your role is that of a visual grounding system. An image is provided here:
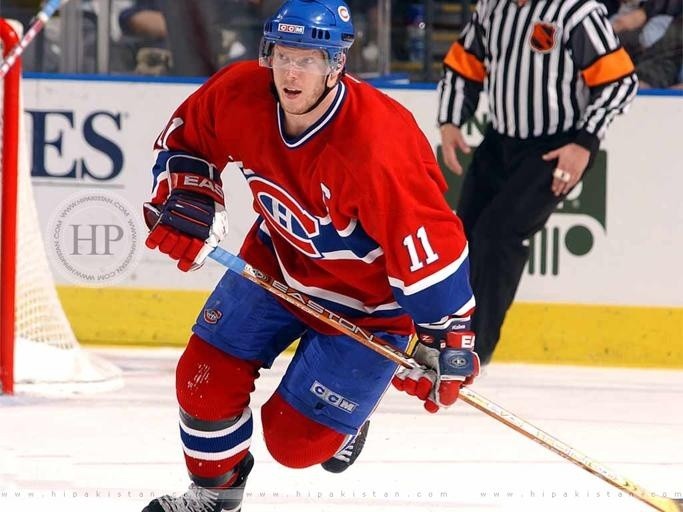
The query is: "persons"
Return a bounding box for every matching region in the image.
[21,0,683,101]
[430,0,639,369]
[123,1,481,511]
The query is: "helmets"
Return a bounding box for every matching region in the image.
[263,1,356,53]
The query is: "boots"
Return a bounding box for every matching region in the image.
[141,452,254,512]
[321,417,371,478]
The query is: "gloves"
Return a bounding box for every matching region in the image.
[141,153,233,275]
[392,304,484,418]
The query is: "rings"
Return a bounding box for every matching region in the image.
[562,173,570,183]
[553,168,565,179]
